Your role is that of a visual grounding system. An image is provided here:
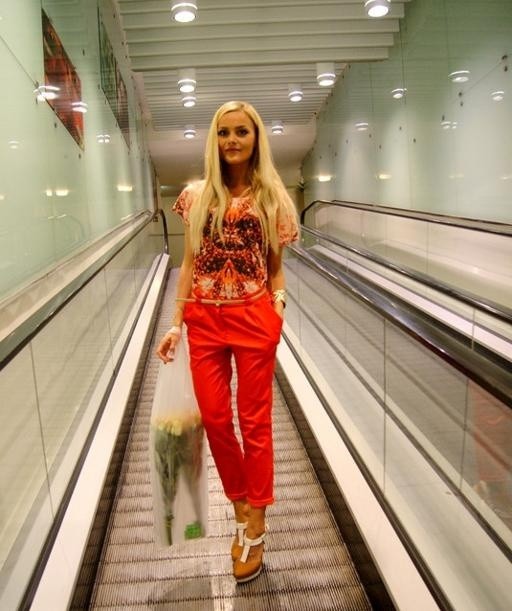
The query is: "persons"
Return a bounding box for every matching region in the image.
[156,101,301,583]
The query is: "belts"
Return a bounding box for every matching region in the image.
[173,288,268,308]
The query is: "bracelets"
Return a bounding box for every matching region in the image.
[270,288,288,308]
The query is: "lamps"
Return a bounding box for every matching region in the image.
[176,68,198,109]
[70,102,89,115]
[448,67,472,86]
[490,90,506,102]
[389,87,408,100]
[363,0,392,18]
[271,119,284,135]
[169,0,198,23]
[95,133,112,146]
[35,84,61,103]
[440,119,460,132]
[288,63,337,103]
[354,121,370,136]
[182,123,197,139]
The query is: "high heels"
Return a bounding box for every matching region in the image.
[231,521,270,584]
